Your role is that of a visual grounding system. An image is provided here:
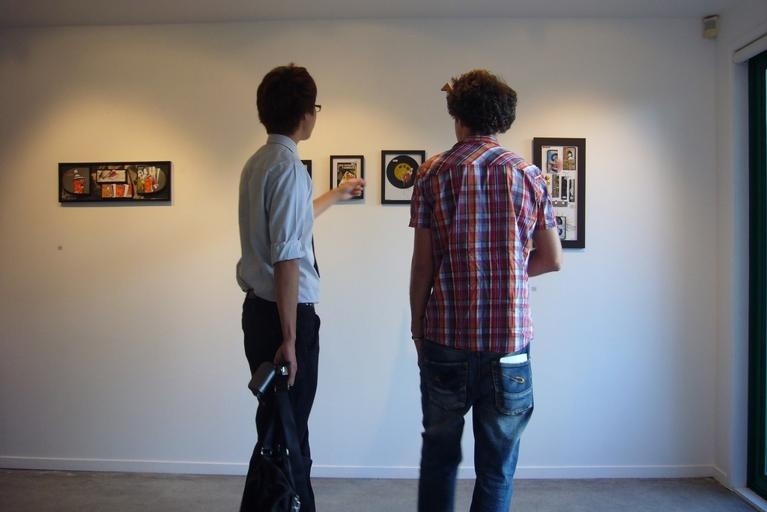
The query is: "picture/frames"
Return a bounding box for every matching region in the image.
[330,155,364,199]
[301,160,312,180]
[533,137,586,248]
[381,150,425,204]
[58,161,173,203]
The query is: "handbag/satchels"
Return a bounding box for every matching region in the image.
[239,442,316,512]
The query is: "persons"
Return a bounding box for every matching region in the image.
[407,67,562,511]
[235,61,367,510]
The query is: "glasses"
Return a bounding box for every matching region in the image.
[310,104,321,111]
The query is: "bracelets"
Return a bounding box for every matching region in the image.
[411,336,423,341]
